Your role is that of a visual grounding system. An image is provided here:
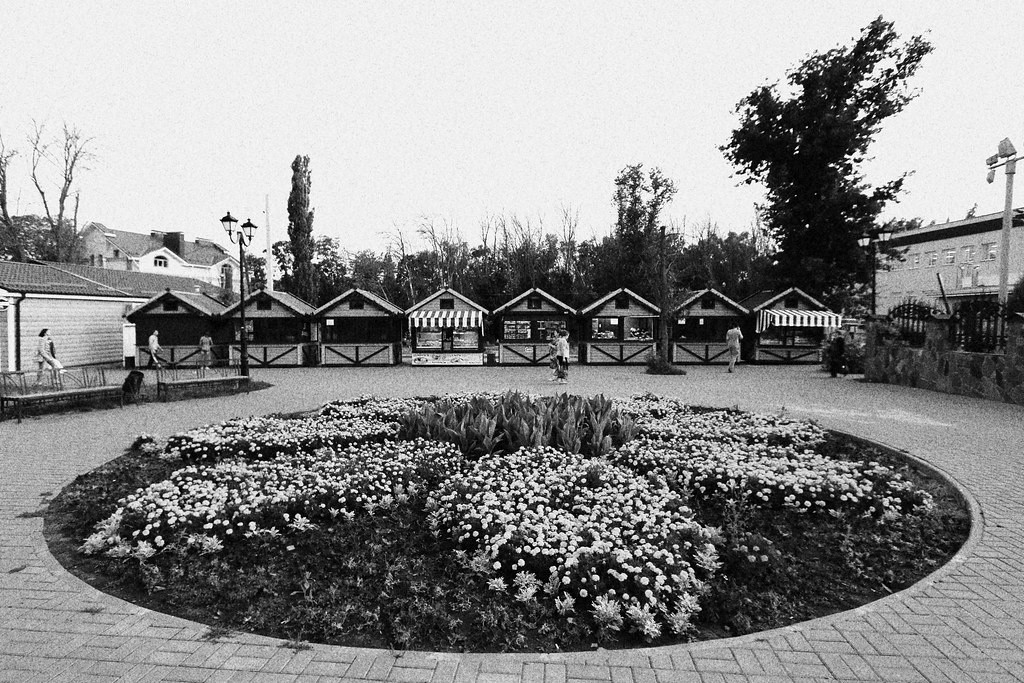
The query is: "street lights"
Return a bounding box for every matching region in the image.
[218,211,257,376]
[984,136,1024,353]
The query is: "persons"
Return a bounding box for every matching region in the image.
[726,322,743,373]
[549,328,570,384]
[829,331,848,378]
[145,330,163,370]
[37,328,68,375]
[199,332,213,369]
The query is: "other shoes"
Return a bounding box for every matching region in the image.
[553,374,558,376]
[205,367,210,370]
[728,368,732,373]
[558,381,567,384]
[201,367,203,369]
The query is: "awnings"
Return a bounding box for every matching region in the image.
[755,307,842,333]
[408,306,485,338]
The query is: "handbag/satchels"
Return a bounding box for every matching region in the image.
[558,370,568,379]
[550,362,558,369]
[33,355,43,363]
